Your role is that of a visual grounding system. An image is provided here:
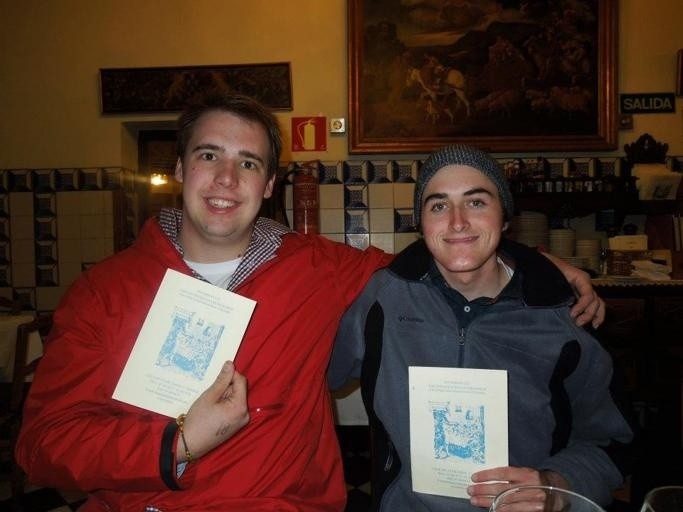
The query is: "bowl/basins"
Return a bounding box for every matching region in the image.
[548,229,601,270]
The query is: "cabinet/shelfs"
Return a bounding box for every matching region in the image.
[514,174,682,471]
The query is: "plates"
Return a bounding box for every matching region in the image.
[507,211,547,250]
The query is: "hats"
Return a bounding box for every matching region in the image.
[412,144,513,230]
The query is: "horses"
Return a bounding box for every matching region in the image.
[406,65,471,124]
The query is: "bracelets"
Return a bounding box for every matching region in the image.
[175,408,193,462]
[542,471,555,511]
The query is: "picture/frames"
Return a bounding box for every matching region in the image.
[348,0,619,155]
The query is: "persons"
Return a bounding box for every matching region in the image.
[15,88,606,512]
[323,141,634,511]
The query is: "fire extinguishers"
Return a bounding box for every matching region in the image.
[285,159,317,235]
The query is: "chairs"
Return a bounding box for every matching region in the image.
[11,317,53,413]
